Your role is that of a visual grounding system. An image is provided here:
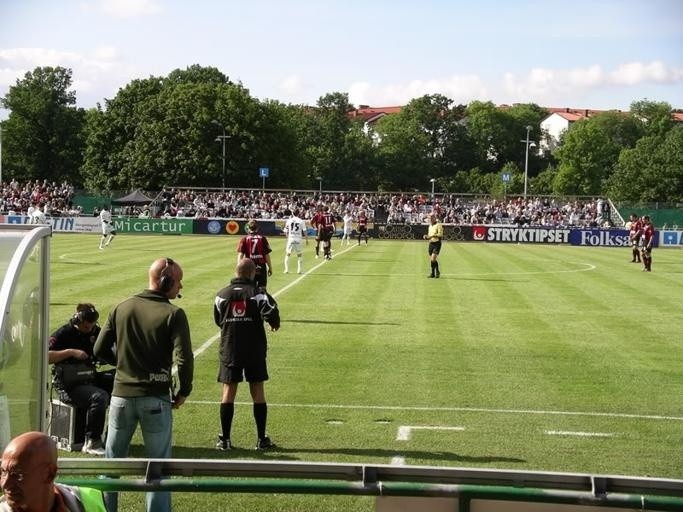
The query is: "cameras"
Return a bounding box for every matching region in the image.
[207,202,214,208]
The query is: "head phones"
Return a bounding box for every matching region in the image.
[157,258,175,292]
[70,308,99,330]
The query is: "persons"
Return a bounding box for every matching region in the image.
[0,431,107,511]
[312,209,326,258]
[506,196,612,228]
[99,205,116,249]
[333,193,427,223]
[94,258,194,512]
[119,205,151,218]
[424,214,444,279]
[316,208,336,261]
[27,200,46,263]
[0,179,83,216]
[341,212,353,247]
[49,303,117,455]
[427,195,506,224]
[158,188,258,219]
[213,258,280,449]
[283,209,309,274]
[237,218,274,290]
[357,211,368,245]
[628,215,641,262]
[257,191,333,219]
[93,207,100,217]
[638,216,655,272]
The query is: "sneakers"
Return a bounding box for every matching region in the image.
[214,433,232,451]
[435,271,440,278]
[81,433,107,457]
[254,435,276,452]
[427,273,435,278]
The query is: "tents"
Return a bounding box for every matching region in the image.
[110,190,156,217]
